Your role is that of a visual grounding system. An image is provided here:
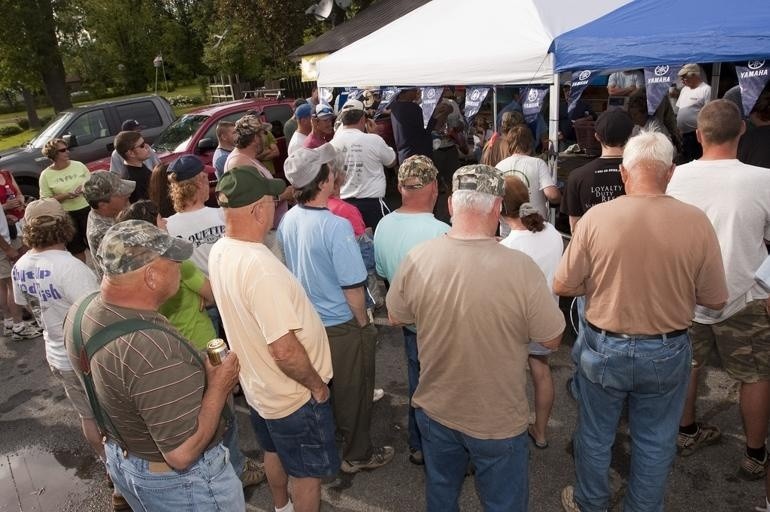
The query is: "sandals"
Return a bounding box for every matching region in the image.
[527,424,548,450]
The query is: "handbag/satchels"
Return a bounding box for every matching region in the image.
[6,217,17,240]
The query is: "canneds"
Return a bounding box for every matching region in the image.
[206,339,229,365]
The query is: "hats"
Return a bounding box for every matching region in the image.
[311,103,335,120]
[452,163,506,197]
[131,140,146,152]
[121,120,140,132]
[232,115,273,141]
[676,62,701,77]
[397,154,440,190]
[96,220,195,275]
[23,198,63,228]
[215,166,285,207]
[283,142,337,190]
[80,171,137,203]
[294,104,313,121]
[165,155,205,182]
[593,109,633,147]
[342,99,368,114]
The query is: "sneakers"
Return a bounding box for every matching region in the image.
[561,484,581,511]
[3,319,43,340]
[409,449,424,466]
[103,470,116,487]
[676,421,721,457]
[371,389,386,402]
[112,494,131,510]
[239,457,268,487]
[339,445,396,473]
[738,445,769,478]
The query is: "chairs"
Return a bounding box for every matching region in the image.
[93,119,109,137]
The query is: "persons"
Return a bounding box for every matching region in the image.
[115,198,267,492]
[496,88,550,155]
[390,87,444,165]
[38,137,91,264]
[60,219,249,512]
[480,110,537,167]
[109,119,162,179]
[563,107,635,404]
[737,94,769,169]
[606,69,645,99]
[385,164,566,512]
[213,120,235,183]
[282,97,308,144]
[329,98,398,314]
[472,116,495,162]
[493,123,562,238]
[304,86,320,114]
[1,203,43,342]
[551,121,730,511]
[497,175,565,449]
[244,109,280,178]
[303,104,335,149]
[543,87,598,132]
[0,170,33,321]
[664,99,769,480]
[113,130,153,206]
[312,142,386,403]
[10,198,131,510]
[418,97,466,216]
[148,160,178,233]
[275,143,395,473]
[666,63,713,165]
[373,155,450,466]
[165,153,245,400]
[223,115,294,261]
[628,87,672,140]
[333,87,351,116]
[81,170,137,281]
[287,103,317,156]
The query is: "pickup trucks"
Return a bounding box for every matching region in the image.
[84,97,394,204]
[1,95,175,207]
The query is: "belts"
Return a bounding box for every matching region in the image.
[586,318,689,340]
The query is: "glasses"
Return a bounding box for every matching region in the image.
[56,146,73,153]
[315,108,333,118]
